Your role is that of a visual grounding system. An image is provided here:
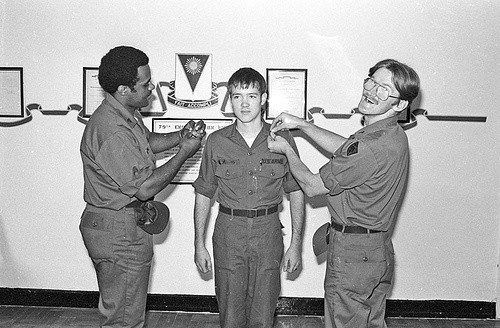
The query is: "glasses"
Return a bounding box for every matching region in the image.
[363,78,401,101]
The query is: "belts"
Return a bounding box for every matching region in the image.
[330,221,388,234]
[218,204,278,218]
[125,196,154,208]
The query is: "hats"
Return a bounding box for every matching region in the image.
[313,222,330,256]
[136,201,170,235]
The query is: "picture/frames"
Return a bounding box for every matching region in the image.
[151,118,233,185]
[83,67,106,118]
[0,67,24,118]
[266,68,308,120]
[397,106,410,123]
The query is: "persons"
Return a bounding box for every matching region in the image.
[192,66,306,327]
[79,45,208,327]
[268,58,419,327]
